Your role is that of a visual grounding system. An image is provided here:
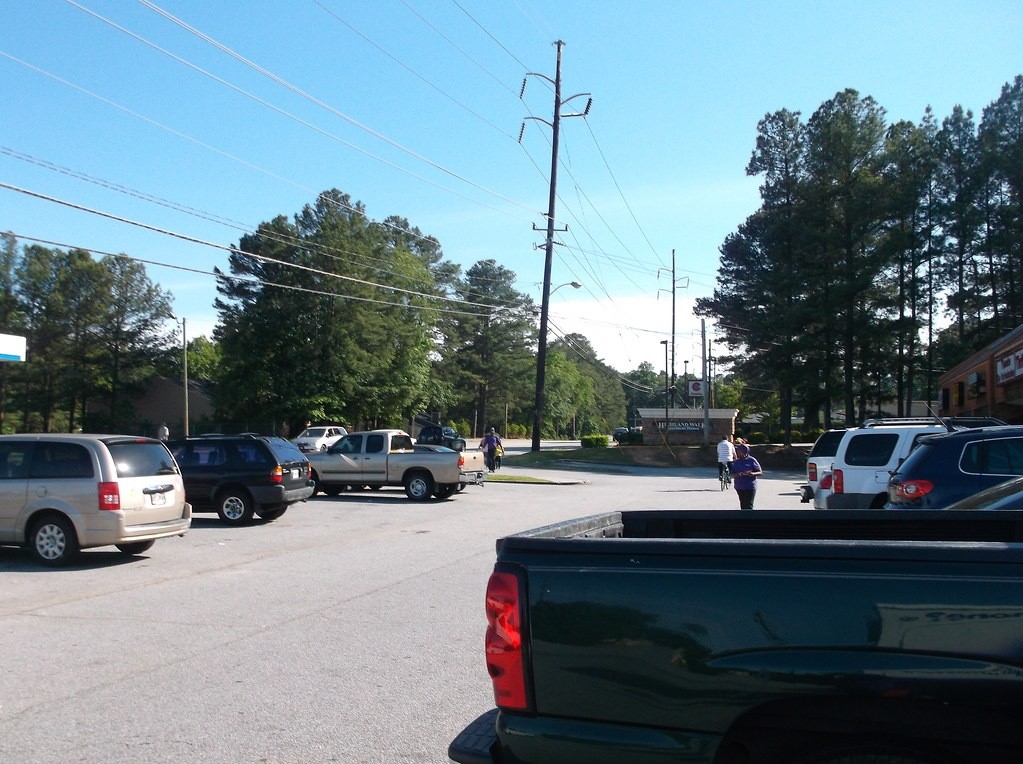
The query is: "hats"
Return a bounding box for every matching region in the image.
[735,445,750,454]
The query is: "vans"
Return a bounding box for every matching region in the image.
[831,424,972,494]
[859,414,1009,432]
[0,436,194,569]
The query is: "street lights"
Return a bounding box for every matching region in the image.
[518,37,592,455]
[661,339,669,446]
[167,311,189,439]
[683,361,689,409]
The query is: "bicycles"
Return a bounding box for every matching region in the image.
[719,462,731,492]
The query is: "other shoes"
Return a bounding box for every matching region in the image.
[719,476,722,481]
[726,477,731,483]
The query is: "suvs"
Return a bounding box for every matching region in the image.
[882,401,1022,510]
[159,433,316,528]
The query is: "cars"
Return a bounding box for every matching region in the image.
[296,426,349,453]
[417,427,467,453]
[810,468,838,510]
[612,425,643,442]
[800,426,869,505]
[939,474,1023,511]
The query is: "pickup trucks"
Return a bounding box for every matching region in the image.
[302,428,485,502]
[486,508,1023,764]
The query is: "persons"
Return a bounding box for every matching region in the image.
[732,444,762,509]
[717,434,737,483]
[476,427,504,473]
[158,422,169,440]
